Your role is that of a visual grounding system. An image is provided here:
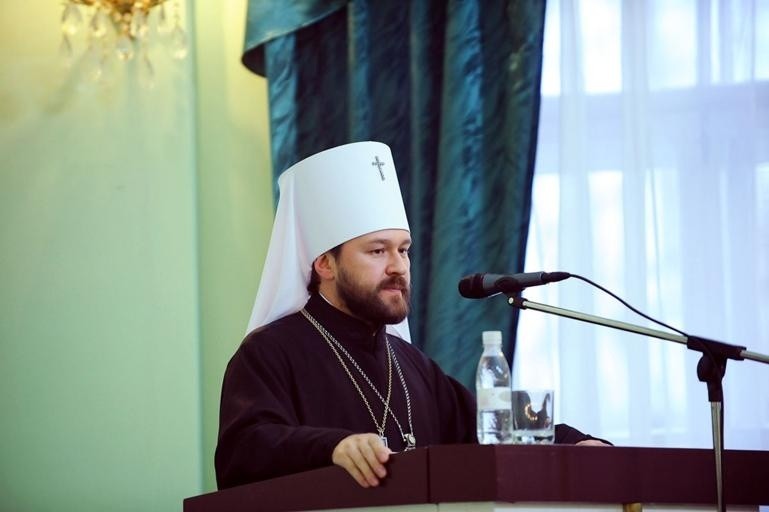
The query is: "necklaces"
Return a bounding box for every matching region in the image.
[300,307,416,455]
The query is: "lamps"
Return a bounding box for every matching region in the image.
[62,0,188,90]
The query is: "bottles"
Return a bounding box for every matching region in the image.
[472,327,512,446]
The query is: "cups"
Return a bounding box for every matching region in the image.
[512,390,556,445]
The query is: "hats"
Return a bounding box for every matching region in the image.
[277,142,412,283]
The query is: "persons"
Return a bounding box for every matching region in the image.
[213,230,615,489]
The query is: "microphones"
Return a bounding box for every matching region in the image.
[457,271,570,299]
